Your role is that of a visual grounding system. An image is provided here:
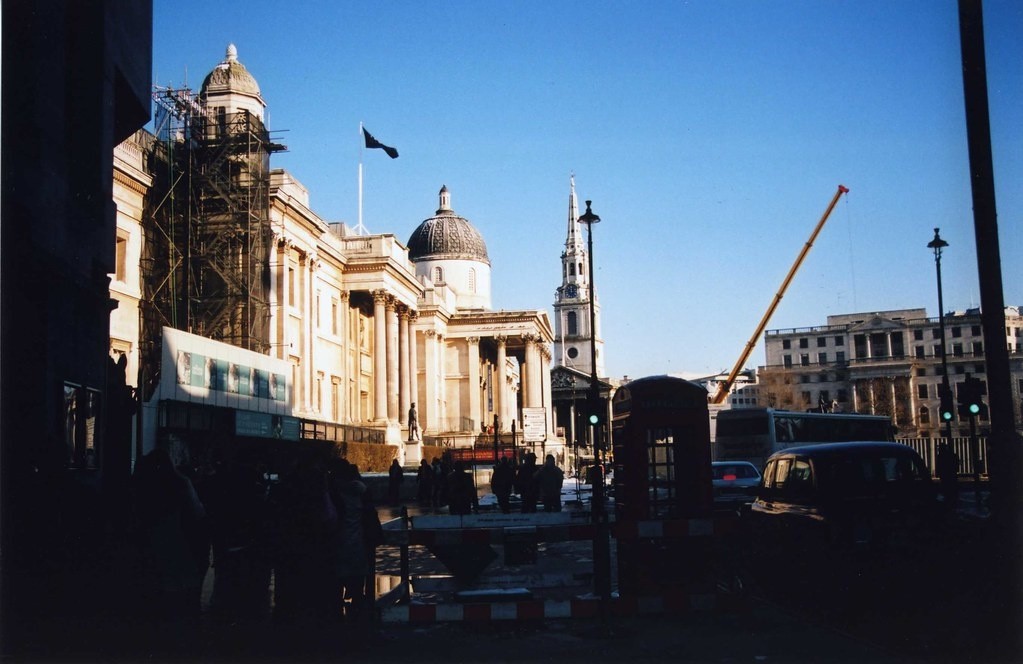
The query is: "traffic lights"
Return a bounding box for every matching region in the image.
[587,397,603,425]
[964,378,983,415]
[939,389,954,423]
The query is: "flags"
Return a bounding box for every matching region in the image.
[362,124,398,158]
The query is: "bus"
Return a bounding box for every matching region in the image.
[715,405,896,478]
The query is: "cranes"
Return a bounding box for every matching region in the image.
[712,183,849,403]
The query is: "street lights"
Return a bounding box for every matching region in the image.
[578,200,605,507]
[927,227,952,446]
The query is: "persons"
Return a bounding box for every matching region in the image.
[409,402,418,441]
[385,461,403,505]
[513,453,563,511]
[144,444,383,606]
[938,441,961,495]
[416,453,479,513]
[491,457,517,514]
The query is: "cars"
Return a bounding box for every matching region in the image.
[735,437,964,616]
[708,459,763,507]
